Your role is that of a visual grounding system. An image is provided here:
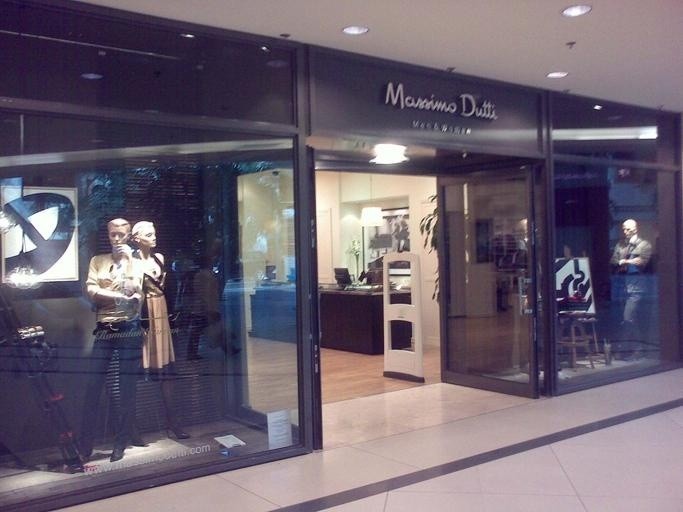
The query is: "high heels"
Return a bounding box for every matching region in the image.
[126,432,148,446]
[165,425,192,438]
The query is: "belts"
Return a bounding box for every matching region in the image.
[93,321,139,333]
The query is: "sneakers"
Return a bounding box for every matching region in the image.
[79,454,91,465]
[110,449,124,462]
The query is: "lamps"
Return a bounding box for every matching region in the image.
[5,114,45,290]
[361,173,384,227]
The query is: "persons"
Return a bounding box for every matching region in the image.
[76,216,145,463]
[128,220,190,447]
[602,218,652,366]
[514,217,562,371]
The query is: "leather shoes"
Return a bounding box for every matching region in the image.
[630,350,644,361]
[615,349,625,361]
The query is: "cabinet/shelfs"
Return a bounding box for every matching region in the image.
[248,283,411,355]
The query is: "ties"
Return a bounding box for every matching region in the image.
[621,245,631,272]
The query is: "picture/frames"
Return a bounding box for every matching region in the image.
[360,206,409,275]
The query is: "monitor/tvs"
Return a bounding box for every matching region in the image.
[334,268,352,287]
[266,266,276,279]
